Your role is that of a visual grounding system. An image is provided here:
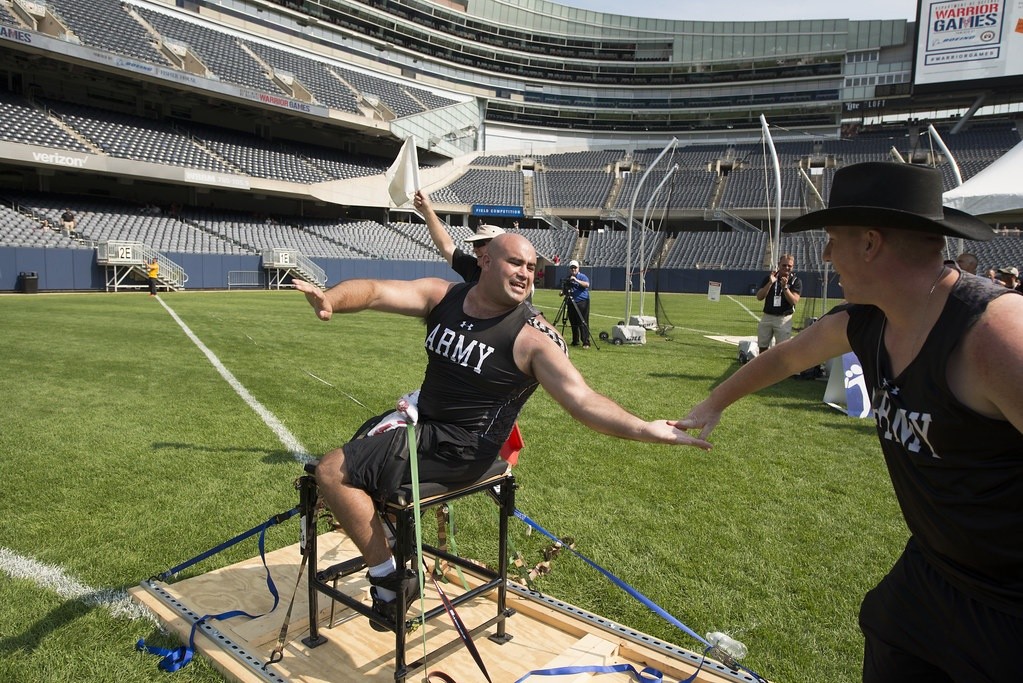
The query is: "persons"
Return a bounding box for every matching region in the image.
[755,254,802,354]
[554,255,559,266]
[667,162,1023,683]
[944,253,1023,295]
[60,208,75,231]
[143,256,160,296]
[41,219,51,228]
[265,217,279,224]
[413,191,532,305]
[290,233,713,633]
[566,260,591,349]
[143,204,162,214]
[537,269,544,288]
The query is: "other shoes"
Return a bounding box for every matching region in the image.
[583,343,591,349]
[568,341,580,346]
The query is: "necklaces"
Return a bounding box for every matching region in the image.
[875,263,946,388]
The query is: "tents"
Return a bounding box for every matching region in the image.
[942,138,1023,225]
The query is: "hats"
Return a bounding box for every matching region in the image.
[569,260,579,268]
[781,161,996,241]
[464,224,505,242]
[153,256,158,260]
[997,266,1018,277]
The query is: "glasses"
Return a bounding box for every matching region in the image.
[570,267,578,269]
[473,241,492,248]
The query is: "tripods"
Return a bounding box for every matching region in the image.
[551,292,600,349]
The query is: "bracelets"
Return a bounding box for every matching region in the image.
[782,287,788,291]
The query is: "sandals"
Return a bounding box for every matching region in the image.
[391,539,416,562]
[366,568,425,633]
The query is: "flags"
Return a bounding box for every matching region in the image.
[383,139,420,207]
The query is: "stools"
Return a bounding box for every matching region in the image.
[301,453,514,683]
